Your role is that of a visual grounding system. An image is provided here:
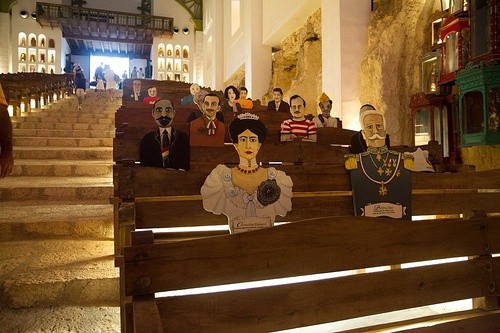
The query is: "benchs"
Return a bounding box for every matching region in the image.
[109,78,500,333]
[0,72,75,117]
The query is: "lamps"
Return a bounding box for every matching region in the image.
[20,10,29,19]
[182,27,190,36]
[30,11,37,20]
[173,27,180,35]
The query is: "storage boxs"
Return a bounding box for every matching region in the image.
[418,51,452,96]
[431,17,443,52]
[449,0,471,17]
[440,0,450,12]
[469,0,500,64]
[440,15,470,84]
[454,61,500,146]
[409,91,463,164]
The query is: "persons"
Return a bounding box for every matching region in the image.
[132,67,138,79]
[122,70,128,78]
[97,75,104,97]
[105,65,115,101]
[75,66,87,110]
[95,62,104,79]
[138,68,144,79]
[0,84,14,178]
